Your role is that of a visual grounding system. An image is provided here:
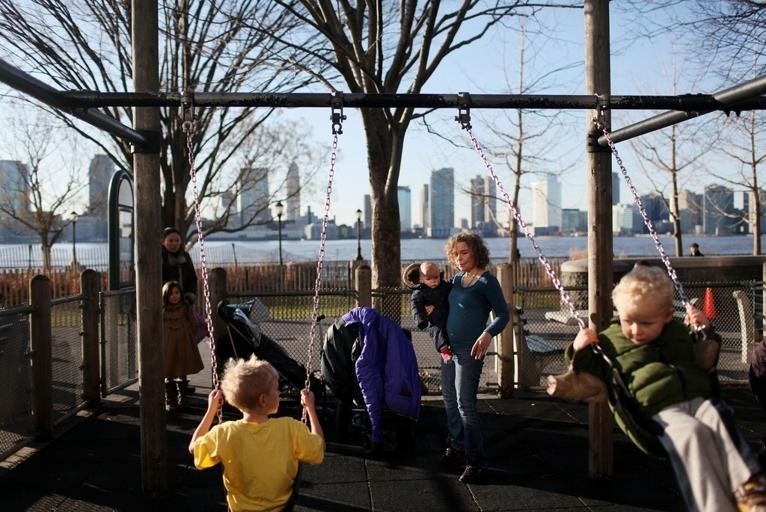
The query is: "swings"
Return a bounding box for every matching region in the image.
[454,92,742,458]
[181,92,347,511]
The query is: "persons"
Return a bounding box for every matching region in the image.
[160,278,204,404]
[563,259,765,512]
[159,224,199,309]
[408,260,453,364]
[687,242,705,257]
[438,227,512,485]
[185,352,326,512]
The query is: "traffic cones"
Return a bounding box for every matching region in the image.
[703,286,720,320]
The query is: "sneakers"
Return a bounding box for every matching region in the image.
[442,447,488,483]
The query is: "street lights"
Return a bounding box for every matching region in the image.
[68,210,80,280]
[275,200,284,264]
[354,208,364,261]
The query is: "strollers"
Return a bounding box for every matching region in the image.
[215,298,330,417]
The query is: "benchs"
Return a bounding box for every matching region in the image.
[521,333,566,378]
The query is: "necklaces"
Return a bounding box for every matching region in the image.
[459,268,482,288]
